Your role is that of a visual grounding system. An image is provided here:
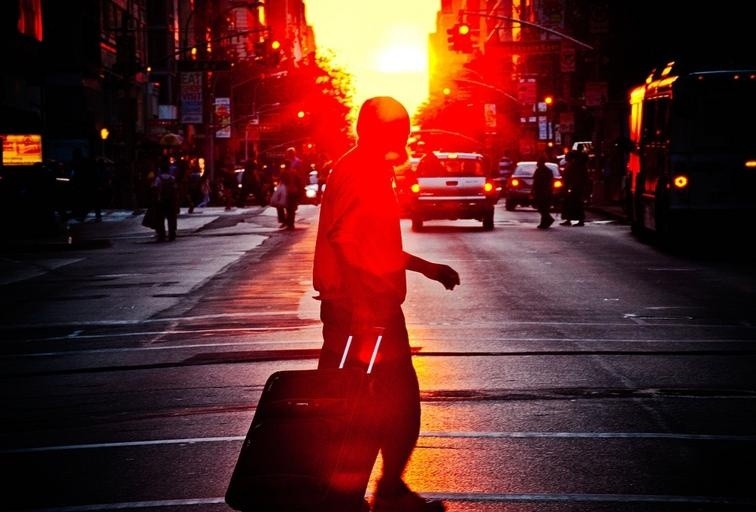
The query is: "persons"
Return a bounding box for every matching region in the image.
[312,96,462,512]
[531,151,591,230]
[146,156,272,243]
[275,147,303,227]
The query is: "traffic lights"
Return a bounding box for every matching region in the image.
[543,94,555,105]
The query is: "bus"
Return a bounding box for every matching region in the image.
[626,60,756,232]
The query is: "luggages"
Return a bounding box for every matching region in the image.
[224,368,388,512]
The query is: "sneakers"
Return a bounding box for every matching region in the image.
[537,216,554,229]
[279,222,295,231]
[156,232,177,244]
[560,221,584,227]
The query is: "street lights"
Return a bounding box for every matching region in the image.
[458,11,594,54]
[133,26,314,153]
[440,77,517,105]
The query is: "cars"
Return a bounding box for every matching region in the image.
[234,169,256,200]
[506,161,565,210]
[411,152,493,231]
[307,170,326,192]
